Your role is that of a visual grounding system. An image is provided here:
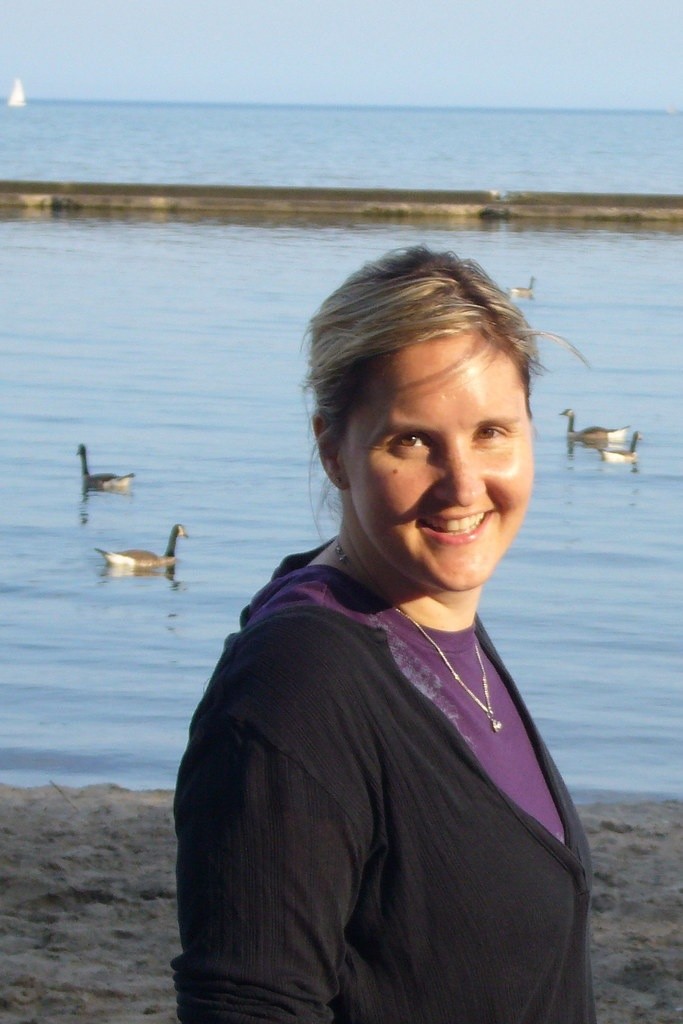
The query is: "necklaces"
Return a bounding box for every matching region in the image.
[335,538,503,733]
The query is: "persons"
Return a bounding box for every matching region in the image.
[168,242,594,1024]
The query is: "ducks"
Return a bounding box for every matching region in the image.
[74,443,136,490]
[95,523,189,567]
[558,407,645,464]
[509,275,537,299]
